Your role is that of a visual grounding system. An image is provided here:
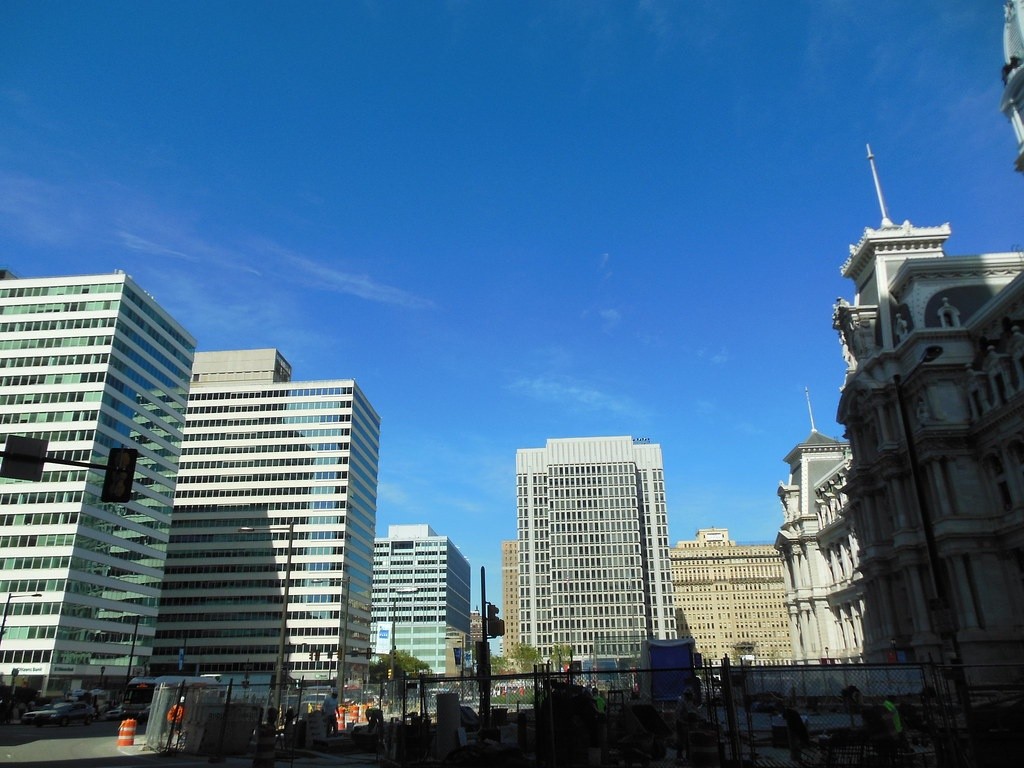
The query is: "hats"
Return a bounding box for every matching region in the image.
[180,696,185,703]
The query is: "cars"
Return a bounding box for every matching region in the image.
[35,701,96,728]
[106,705,123,721]
[21,703,51,725]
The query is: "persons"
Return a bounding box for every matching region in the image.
[676,678,702,760]
[882,694,914,754]
[590,688,608,719]
[253,707,280,768]
[322,689,339,738]
[166,695,186,736]
[775,687,810,762]
[366,708,384,734]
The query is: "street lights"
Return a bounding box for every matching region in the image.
[236,522,294,748]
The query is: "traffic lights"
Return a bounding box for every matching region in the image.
[308,647,343,661]
[367,648,372,660]
[100,448,137,503]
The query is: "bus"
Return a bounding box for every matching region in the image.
[121,676,157,725]
